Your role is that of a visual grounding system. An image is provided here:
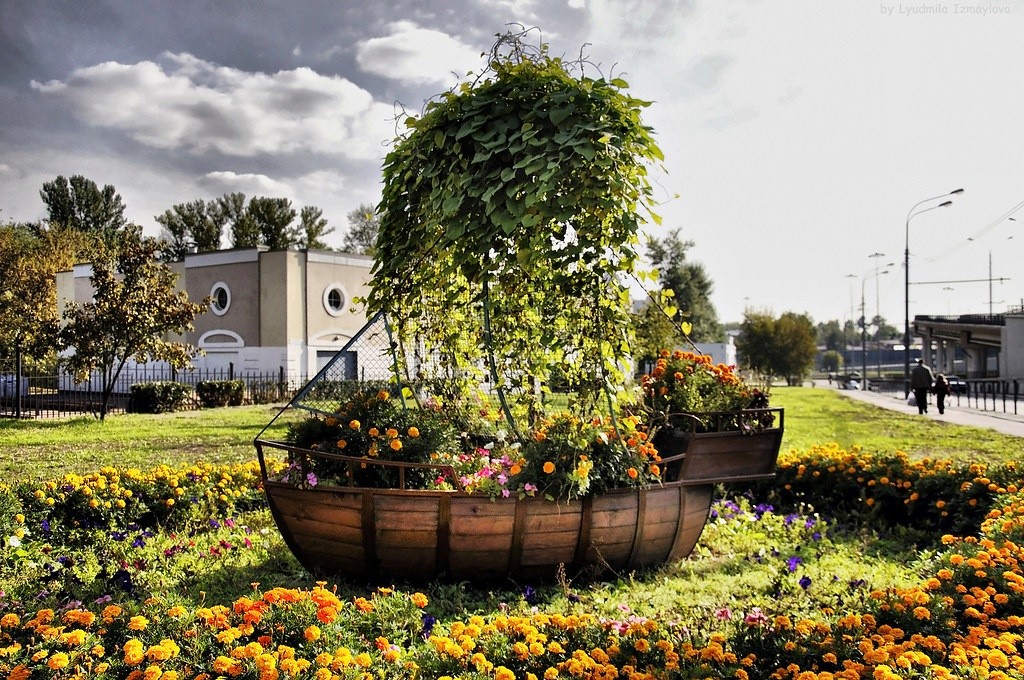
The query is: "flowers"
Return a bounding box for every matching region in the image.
[266,349,776,506]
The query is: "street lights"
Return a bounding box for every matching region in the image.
[905,188,964,399]
[968,235,1014,325]
[861,262,895,390]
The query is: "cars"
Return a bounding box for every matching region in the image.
[843,371,871,391]
[931,375,968,394]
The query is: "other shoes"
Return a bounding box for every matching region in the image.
[919,411,923,414]
[925,410,927,413]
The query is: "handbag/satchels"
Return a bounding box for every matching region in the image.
[907,391,917,406]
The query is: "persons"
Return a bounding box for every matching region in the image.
[933,374,951,415]
[910,360,933,414]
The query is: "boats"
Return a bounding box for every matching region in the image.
[252,63,784,587]
